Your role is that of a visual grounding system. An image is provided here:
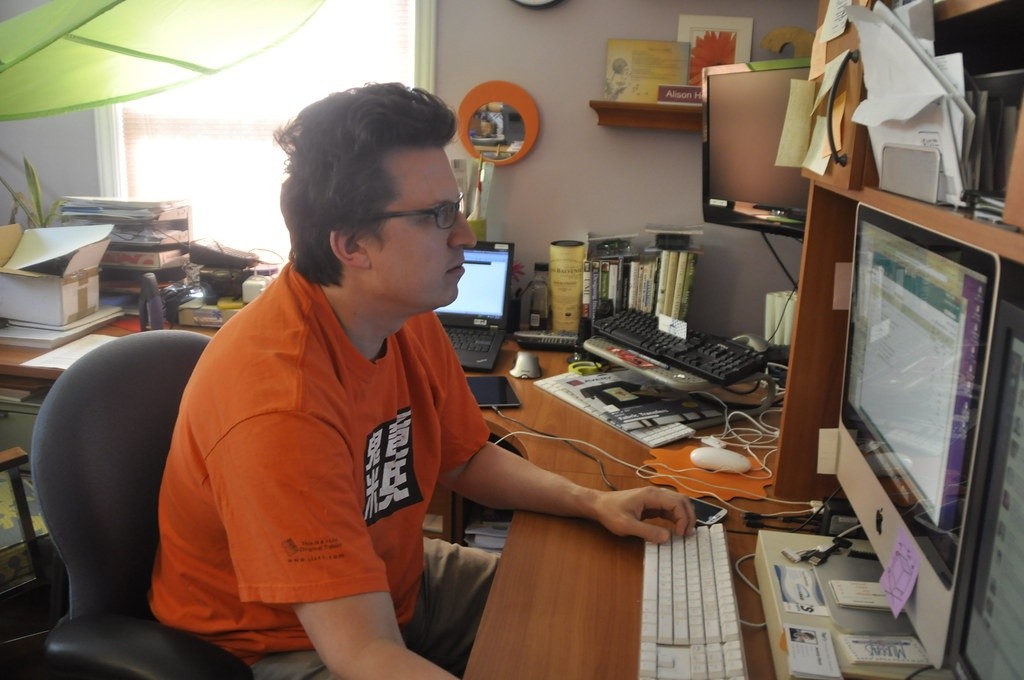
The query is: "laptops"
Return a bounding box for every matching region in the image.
[433,240,515,372]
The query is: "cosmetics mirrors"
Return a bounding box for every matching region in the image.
[458,81,539,165]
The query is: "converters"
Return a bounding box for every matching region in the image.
[820,497,869,541]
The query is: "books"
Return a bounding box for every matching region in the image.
[582,249,698,324]
[0,379,53,402]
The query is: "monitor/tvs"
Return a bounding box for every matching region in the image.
[812,201,1011,668]
[701,57,813,238]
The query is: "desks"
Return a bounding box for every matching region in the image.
[0,314,820,680]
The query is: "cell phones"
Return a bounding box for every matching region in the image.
[658,487,728,526]
[466,376,521,408]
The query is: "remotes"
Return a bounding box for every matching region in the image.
[513,331,588,353]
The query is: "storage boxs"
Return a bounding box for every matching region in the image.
[0,224,110,326]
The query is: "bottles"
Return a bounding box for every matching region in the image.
[527,262,551,332]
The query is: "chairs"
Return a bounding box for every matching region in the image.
[0,447,63,662]
[42,330,255,680]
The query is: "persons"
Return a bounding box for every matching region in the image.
[149,82,696,680]
[790,628,814,642]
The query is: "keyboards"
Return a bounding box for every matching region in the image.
[593,307,766,385]
[638,523,749,680]
[532,371,696,447]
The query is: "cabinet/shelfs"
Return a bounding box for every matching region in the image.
[771,0,1024,497]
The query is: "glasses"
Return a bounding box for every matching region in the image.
[353,192,465,230]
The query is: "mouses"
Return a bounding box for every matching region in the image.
[690,448,752,473]
[508,350,541,378]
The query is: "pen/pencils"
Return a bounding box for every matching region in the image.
[627,349,671,371]
